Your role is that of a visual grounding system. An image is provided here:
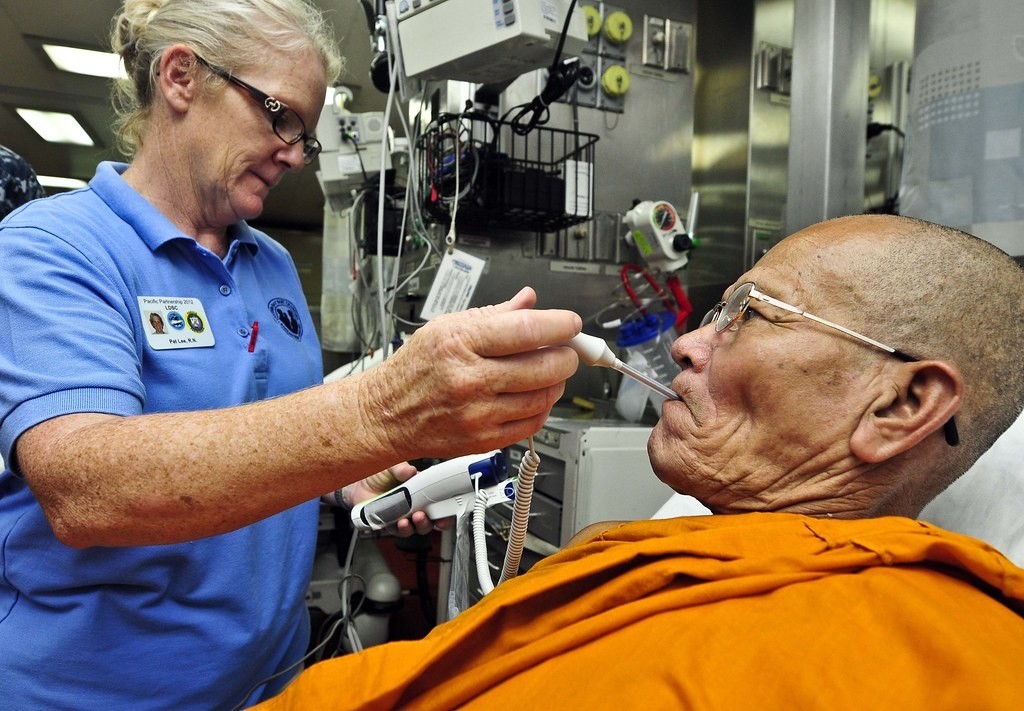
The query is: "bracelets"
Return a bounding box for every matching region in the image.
[333,489,351,515]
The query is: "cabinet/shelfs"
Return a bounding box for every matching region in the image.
[467,415,676,608]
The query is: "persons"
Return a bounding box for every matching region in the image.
[242,214,1024,711]
[0,0,582,711]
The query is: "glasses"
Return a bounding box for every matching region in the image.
[188,53,322,165]
[700,282,964,446]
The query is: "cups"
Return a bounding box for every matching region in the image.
[615,310,685,418]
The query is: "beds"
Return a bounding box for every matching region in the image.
[650,408,1024,572]
